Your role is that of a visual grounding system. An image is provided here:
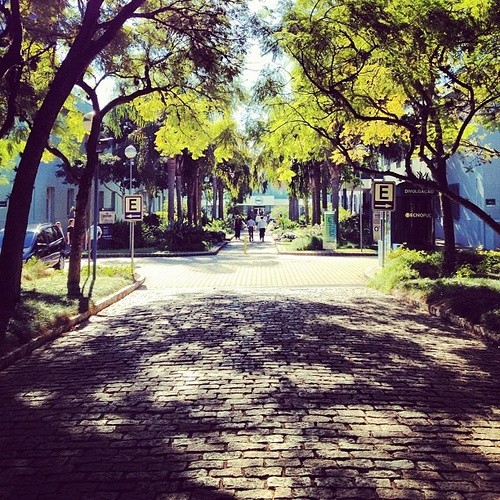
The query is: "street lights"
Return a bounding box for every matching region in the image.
[83,111,102,280]
[204,177,209,225]
[123,144,138,276]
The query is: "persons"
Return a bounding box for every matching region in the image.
[87,220,102,262]
[234,215,243,240]
[65,218,74,259]
[258,217,267,243]
[55,221,65,242]
[247,215,256,242]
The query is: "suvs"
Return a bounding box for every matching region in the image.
[2,224,69,270]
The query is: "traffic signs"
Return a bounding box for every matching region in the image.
[123,196,143,222]
[372,181,396,212]
